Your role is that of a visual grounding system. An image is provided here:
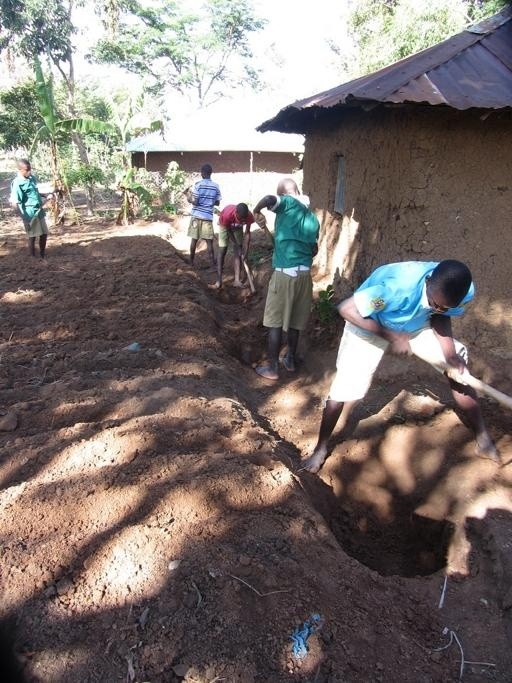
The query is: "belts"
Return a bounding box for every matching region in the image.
[275,265,309,277]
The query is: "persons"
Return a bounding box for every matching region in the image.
[299,258,505,471]
[216,200,255,289]
[251,178,320,380]
[9,159,50,257]
[186,164,221,264]
